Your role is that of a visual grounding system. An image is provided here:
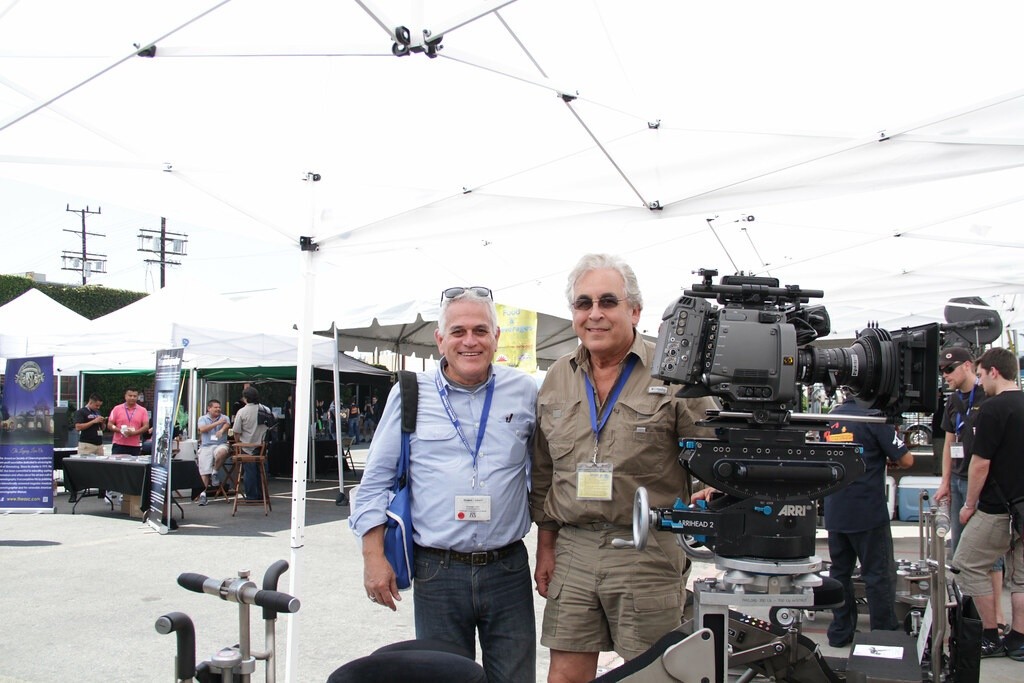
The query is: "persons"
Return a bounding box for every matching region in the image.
[233,386,271,502]
[346,287,541,683]
[932,345,1011,639]
[107,388,149,456]
[952,347,1024,661]
[143,418,153,456]
[197,399,231,506]
[73,392,106,455]
[819,389,915,648]
[231,383,250,422]
[285,393,383,446]
[528,254,722,683]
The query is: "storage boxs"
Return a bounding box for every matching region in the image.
[130,495,143,518]
[885,476,897,521]
[120,500,130,514]
[122,494,130,501]
[897,474,942,522]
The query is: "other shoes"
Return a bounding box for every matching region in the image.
[828,628,856,648]
[360,439,365,442]
[68,492,77,502]
[368,439,372,443]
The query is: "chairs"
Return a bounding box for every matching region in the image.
[326,435,357,476]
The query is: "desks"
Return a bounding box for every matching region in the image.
[61,457,207,521]
[53,451,78,493]
[268,439,348,475]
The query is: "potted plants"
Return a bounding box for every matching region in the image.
[62,398,79,447]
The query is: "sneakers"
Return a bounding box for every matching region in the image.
[118,494,123,502]
[210,473,219,486]
[1004,634,1024,661]
[198,496,208,506]
[105,489,113,504]
[981,637,1006,658]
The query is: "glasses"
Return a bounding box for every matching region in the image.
[939,362,964,376]
[571,294,630,311]
[440,287,493,309]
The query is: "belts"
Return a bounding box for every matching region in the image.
[413,540,524,566]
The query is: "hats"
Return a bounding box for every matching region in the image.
[939,348,973,366]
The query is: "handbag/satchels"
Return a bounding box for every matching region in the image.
[257,404,274,427]
[381,368,417,593]
[1008,496,1024,544]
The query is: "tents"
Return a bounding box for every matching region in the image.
[58,284,396,483]
[312,295,578,506]
[0,288,91,414]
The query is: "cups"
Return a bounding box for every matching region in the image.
[121,425,127,432]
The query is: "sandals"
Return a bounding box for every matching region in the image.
[997,622,1010,640]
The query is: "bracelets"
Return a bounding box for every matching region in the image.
[964,501,974,512]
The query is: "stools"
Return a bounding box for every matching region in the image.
[212,440,246,503]
[231,440,273,517]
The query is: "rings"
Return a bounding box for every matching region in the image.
[368,594,376,602]
